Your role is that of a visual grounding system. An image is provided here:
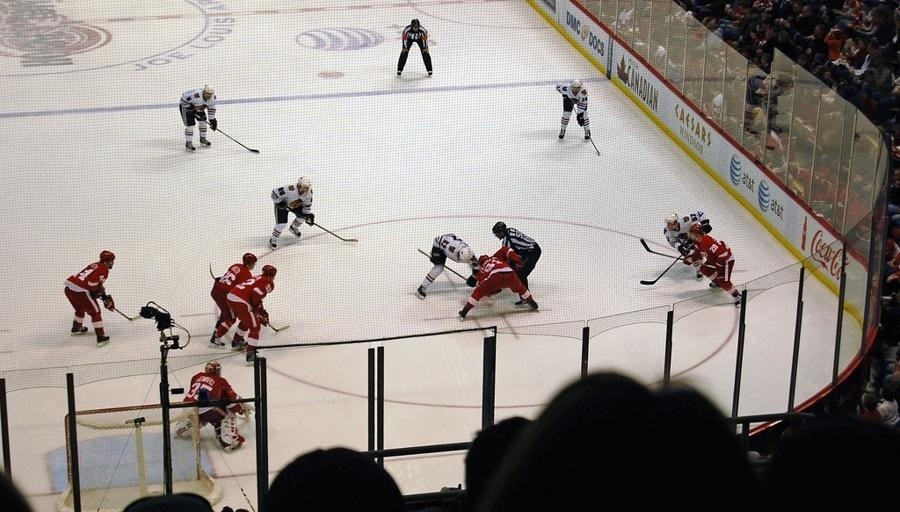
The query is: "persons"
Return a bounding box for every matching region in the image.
[584,0,900,358]
[556,81,594,141]
[211,253,277,363]
[413,219,542,319]
[62,248,116,344]
[178,84,217,150]
[268,178,315,248]
[396,17,434,78]
[1,359,900,512]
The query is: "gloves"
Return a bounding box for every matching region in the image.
[577,113,584,126]
[402,48,408,55]
[466,277,476,286]
[678,245,690,256]
[423,48,429,55]
[700,220,712,233]
[279,201,287,209]
[430,255,441,264]
[306,214,314,226]
[104,295,115,312]
[209,118,217,131]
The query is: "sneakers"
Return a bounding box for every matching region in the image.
[211,334,225,346]
[72,327,88,332]
[290,225,301,236]
[270,239,276,247]
[232,341,248,347]
[459,310,467,318]
[98,336,109,343]
[186,142,195,150]
[735,294,742,305]
[397,71,401,75]
[697,274,702,278]
[428,71,432,75]
[530,301,538,310]
[559,130,565,139]
[200,137,211,145]
[417,285,426,297]
[710,282,718,287]
[585,130,590,139]
[246,352,256,362]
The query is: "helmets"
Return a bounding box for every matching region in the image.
[573,80,582,87]
[479,255,489,266]
[665,212,679,224]
[100,251,115,262]
[492,222,506,233]
[460,247,474,262]
[243,253,257,265]
[298,176,310,187]
[689,224,703,238]
[205,86,215,93]
[205,363,221,375]
[411,19,419,28]
[262,265,277,275]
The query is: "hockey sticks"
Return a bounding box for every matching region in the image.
[640,240,695,285]
[209,261,220,281]
[259,313,290,332]
[567,99,601,156]
[639,238,717,269]
[286,204,358,242]
[193,114,260,152]
[98,296,142,321]
